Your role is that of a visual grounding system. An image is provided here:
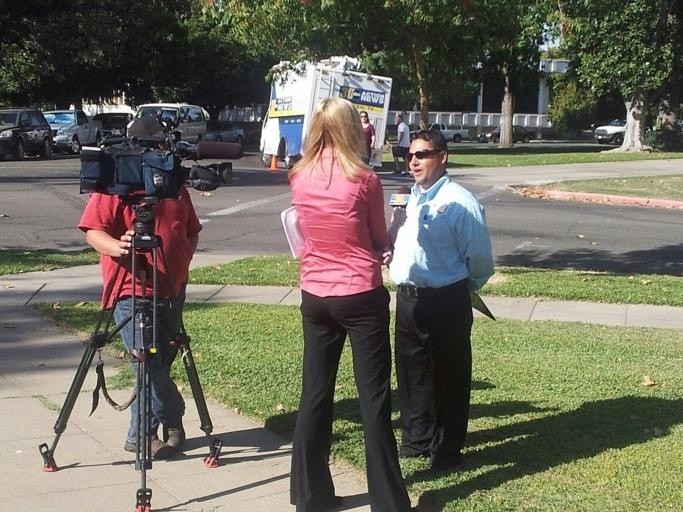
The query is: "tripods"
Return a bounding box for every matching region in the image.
[38,235,223,512]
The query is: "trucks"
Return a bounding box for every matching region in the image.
[258,60,392,170]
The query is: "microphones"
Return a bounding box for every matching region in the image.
[389,186,411,209]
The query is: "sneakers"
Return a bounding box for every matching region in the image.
[391,169,410,177]
[124,434,174,459]
[162,418,185,451]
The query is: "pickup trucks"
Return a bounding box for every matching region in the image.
[92,110,133,145]
[41,109,105,155]
[407,122,469,143]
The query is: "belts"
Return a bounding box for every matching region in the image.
[400,285,439,297]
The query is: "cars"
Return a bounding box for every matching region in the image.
[477,125,536,144]
[203,120,245,149]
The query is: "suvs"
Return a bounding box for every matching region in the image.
[0,105,55,160]
[594,119,648,146]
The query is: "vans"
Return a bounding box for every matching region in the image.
[125,104,207,152]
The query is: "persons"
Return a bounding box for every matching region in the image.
[77,176,203,457]
[285,96,412,511]
[388,129,496,469]
[392,114,410,174]
[358,111,375,164]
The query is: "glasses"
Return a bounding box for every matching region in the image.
[406,150,441,161]
